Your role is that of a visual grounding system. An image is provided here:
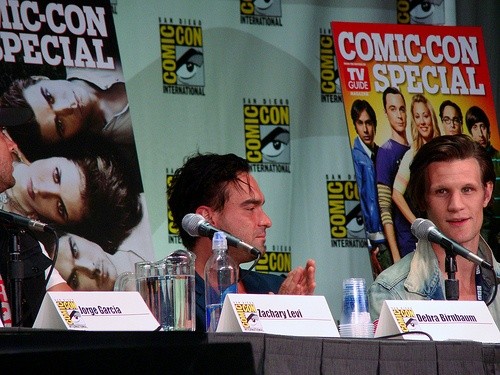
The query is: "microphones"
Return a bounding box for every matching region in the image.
[0,209,55,234]
[411,218,493,270]
[182,213,262,257]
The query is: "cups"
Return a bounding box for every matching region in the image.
[338,278,375,338]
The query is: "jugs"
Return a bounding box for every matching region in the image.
[114,249,197,331]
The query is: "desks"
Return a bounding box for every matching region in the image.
[0,327,500,375]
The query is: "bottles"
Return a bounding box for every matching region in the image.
[203,232,238,333]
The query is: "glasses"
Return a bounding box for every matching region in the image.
[442,118,461,126]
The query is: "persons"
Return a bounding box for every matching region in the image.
[368,134,500,330]
[350,87,500,339]
[125,147,318,331]
[0,58,152,292]
